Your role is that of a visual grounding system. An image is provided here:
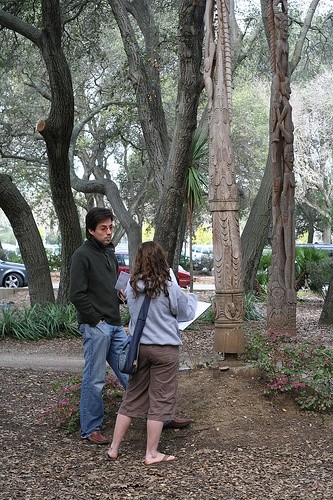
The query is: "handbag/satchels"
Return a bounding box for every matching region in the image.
[116,335,141,375]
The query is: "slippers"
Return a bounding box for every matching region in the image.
[144,453,176,464]
[106,449,116,460]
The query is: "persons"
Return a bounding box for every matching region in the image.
[107,241,197,465]
[68,207,191,445]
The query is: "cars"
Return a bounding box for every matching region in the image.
[1,257,26,290]
[111,249,195,291]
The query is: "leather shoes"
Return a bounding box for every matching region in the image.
[88,432,109,445]
[173,417,189,427]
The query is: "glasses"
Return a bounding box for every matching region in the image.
[95,225,112,231]
